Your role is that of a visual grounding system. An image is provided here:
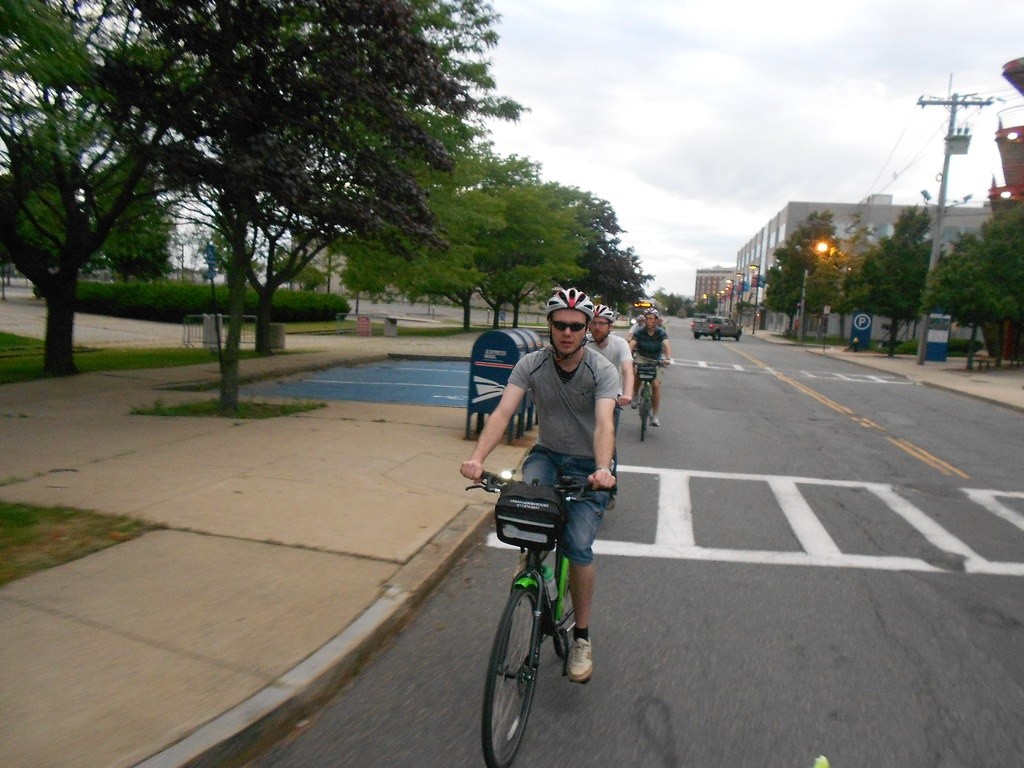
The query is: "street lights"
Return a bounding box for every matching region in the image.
[726,279,734,315]
[749,264,761,334]
[703,290,727,316]
[736,272,745,326]
[815,241,829,254]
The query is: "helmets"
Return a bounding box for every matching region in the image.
[636,315,646,322]
[643,308,659,321]
[544,288,595,320]
[593,303,615,323]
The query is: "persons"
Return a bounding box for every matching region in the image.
[587,303,634,511]
[460,288,619,683]
[627,308,672,426]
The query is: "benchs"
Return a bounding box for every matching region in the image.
[974,358,997,369]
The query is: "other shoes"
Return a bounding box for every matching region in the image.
[605,495,615,511]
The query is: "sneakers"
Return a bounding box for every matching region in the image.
[566,635,593,682]
[513,553,530,578]
[631,394,639,409]
[651,417,659,426]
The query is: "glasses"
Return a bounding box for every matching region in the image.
[548,318,587,331]
[591,320,610,326]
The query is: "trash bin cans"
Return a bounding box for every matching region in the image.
[384,318,397,337]
[464,328,544,444]
[269,324,285,349]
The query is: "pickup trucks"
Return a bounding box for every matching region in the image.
[692,316,743,341]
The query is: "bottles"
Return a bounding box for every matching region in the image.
[643,385,651,399]
[542,564,558,601]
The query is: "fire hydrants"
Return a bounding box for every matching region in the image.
[853,337,860,352]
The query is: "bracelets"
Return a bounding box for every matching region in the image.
[596,467,610,473]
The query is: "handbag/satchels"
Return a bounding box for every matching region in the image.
[494,480,569,550]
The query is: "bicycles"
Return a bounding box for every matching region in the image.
[634,360,666,441]
[459,464,618,768]
